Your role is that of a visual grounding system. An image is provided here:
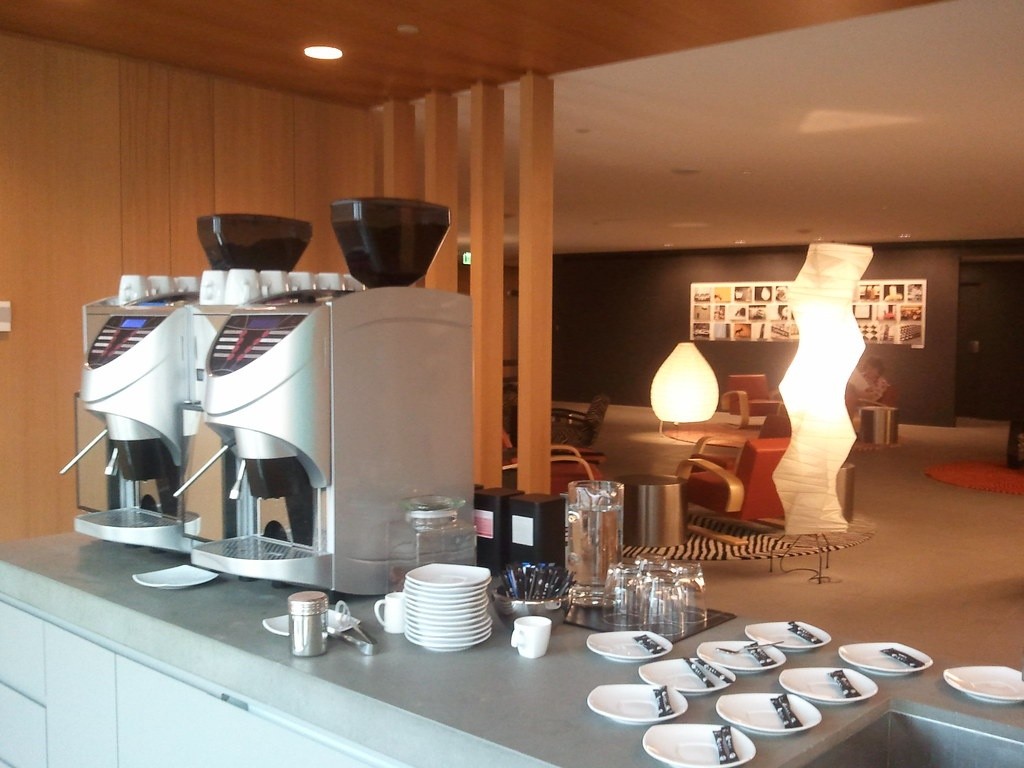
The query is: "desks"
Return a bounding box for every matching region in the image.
[860,406,899,443]
[837,463,855,522]
[612,473,685,546]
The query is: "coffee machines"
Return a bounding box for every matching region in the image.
[59,213,313,556]
[190,196,476,597]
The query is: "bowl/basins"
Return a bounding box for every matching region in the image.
[491,586,571,632]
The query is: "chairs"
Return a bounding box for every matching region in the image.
[501,374,791,544]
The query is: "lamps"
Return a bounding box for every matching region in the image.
[651,342,719,437]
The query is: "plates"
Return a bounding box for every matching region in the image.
[132,565,220,589]
[262,609,361,636]
[942,665,1024,704]
[402,563,494,652]
[585,621,933,768]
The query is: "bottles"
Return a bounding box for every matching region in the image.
[287,590,330,657]
[388,496,479,591]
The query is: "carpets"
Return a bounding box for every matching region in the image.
[925,460,1024,495]
[665,424,902,453]
[622,512,874,561]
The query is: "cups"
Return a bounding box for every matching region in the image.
[603,553,708,636]
[566,480,625,605]
[510,616,552,659]
[374,592,406,634]
[117,268,367,308]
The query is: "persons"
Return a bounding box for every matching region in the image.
[853,358,889,403]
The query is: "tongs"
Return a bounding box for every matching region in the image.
[326,600,379,654]
